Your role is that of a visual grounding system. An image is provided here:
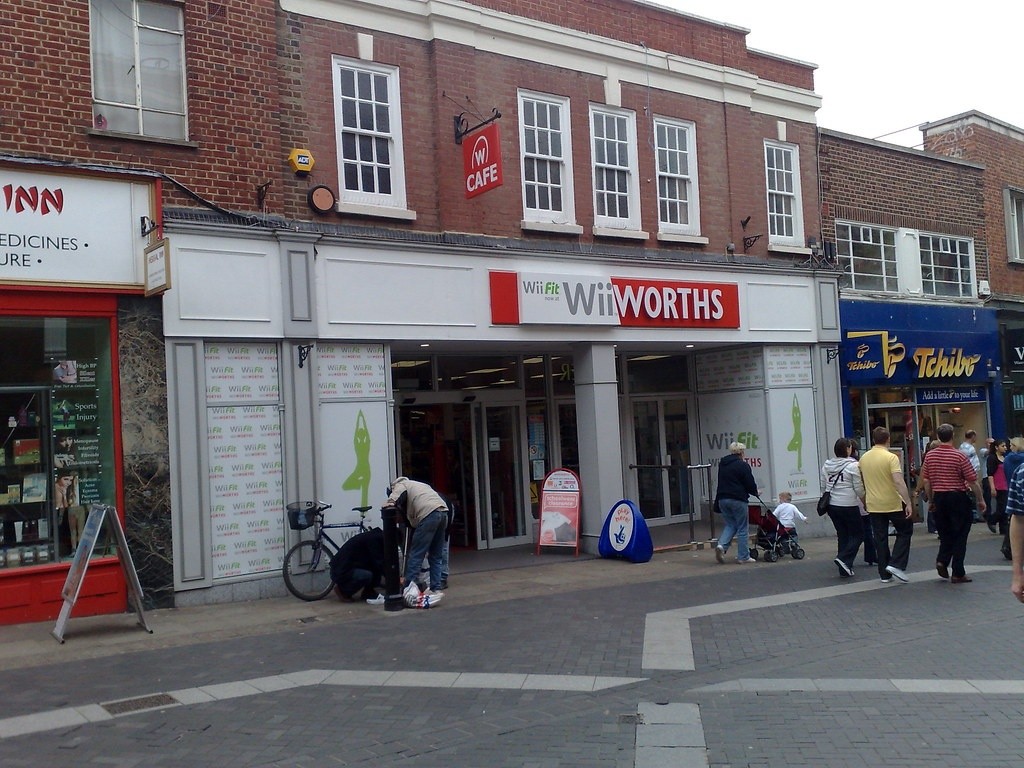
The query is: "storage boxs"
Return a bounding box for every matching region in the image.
[12,439,40,455]
[12,455,41,464]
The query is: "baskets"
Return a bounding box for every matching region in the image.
[287,501,316,530]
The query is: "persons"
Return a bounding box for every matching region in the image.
[986,439,1009,536]
[1004,437,1024,483]
[715,442,761,562]
[979,437,995,518]
[383,476,453,593]
[328,526,405,604]
[821,437,878,576]
[916,423,986,582]
[959,429,985,524]
[56,436,73,454]
[859,426,914,583]
[55,470,75,508]
[1005,462,1024,604]
[772,491,808,531]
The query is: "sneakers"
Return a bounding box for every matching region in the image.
[833,558,855,578]
[886,566,908,581]
[881,577,894,583]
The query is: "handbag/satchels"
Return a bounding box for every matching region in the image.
[817,491,830,516]
[713,493,722,513]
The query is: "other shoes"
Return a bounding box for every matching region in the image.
[952,574,973,583]
[361,589,378,600]
[987,518,997,533]
[384,602,404,611]
[1001,547,1012,560]
[440,581,448,590]
[334,586,354,602]
[929,529,938,533]
[738,557,756,563]
[715,545,725,563]
[936,562,949,578]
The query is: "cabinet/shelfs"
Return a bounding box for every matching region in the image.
[0,388,52,549]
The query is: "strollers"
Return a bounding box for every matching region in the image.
[748,496,805,563]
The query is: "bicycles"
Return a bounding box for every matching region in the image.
[281,498,374,602]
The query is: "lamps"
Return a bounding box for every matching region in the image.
[952,405,961,413]
